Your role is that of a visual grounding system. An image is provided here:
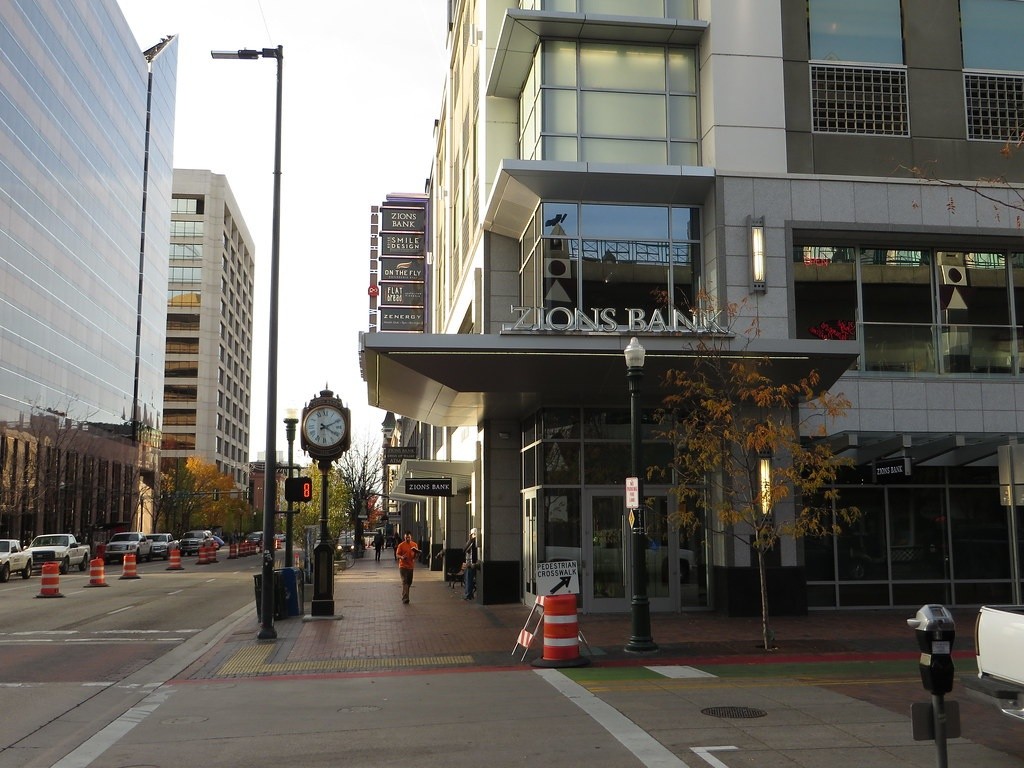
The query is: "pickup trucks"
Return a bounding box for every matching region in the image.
[0,540,34,583]
[146,533,181,560]
[23,533,91,574]
[104,532,153,564]
[545,529,695,586]
[959,605,1024,721]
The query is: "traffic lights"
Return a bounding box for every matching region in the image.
[285,477,312,502]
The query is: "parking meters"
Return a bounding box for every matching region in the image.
[908,604,957,768]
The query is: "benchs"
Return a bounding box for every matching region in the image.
[447,561,468,589]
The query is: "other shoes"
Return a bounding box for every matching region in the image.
[466,596,471,600]
[405,600,409,604]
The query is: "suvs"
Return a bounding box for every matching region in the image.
[180,530,214,557]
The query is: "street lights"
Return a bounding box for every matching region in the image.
[60,482,65,534]
[355,483,360,551]
[211,45,283,643]
[624,337,659,652]
[283,408,300,568]
[254,512,256,532]
[140,496,144,532]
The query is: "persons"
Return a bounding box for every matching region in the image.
[374,529,385,561]
[393,532,402,561]
[396,531,422,603]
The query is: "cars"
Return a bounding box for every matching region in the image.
[364,532,378,547]
[277,534,286,542]
[336,537,354,552]
[245,531,263,543]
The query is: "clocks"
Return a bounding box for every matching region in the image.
[303,406,349,450]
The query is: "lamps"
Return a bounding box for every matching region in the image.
[759,445,774,515]
[746,215,769,295]
